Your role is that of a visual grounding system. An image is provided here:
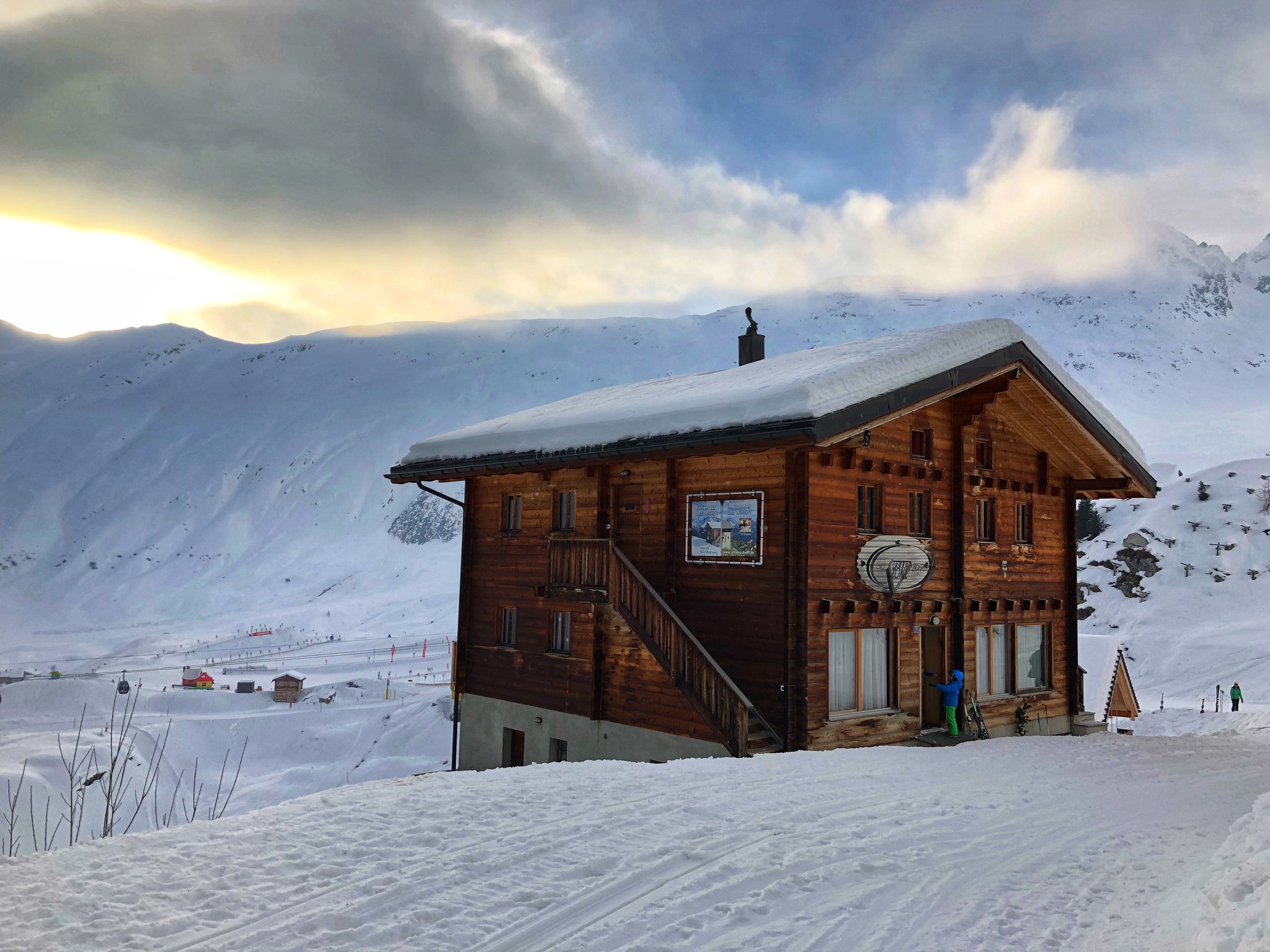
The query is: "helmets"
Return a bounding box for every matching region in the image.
[952,670,964,681]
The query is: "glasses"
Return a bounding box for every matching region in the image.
[949,671,953,676]
[1234,683,1238,685]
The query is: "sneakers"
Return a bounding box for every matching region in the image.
[946,731,949,733]
[945,732,958,738]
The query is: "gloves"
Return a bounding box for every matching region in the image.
[1241,700,1243,703]
[1232,699,1234,701]
[929,683,936,687]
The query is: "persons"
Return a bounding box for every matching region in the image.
[1230,682,1243,712]
[929,670,964,737]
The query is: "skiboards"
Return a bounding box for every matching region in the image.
[963,702,974,735]
[971,696,988,740]
